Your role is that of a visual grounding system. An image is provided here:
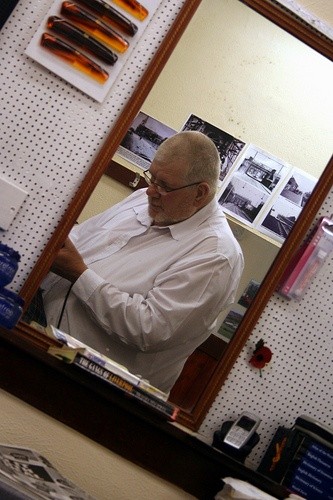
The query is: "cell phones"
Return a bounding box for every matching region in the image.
[223,410,261,449]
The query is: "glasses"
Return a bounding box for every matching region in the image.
[143,170,206,196]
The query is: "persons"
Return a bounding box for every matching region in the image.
[34,131,244,394]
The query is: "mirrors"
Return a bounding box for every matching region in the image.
[17,0,333,434]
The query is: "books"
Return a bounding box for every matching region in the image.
[47,345,178,421]
[31,321,129,371]
[257,426,332,500]
